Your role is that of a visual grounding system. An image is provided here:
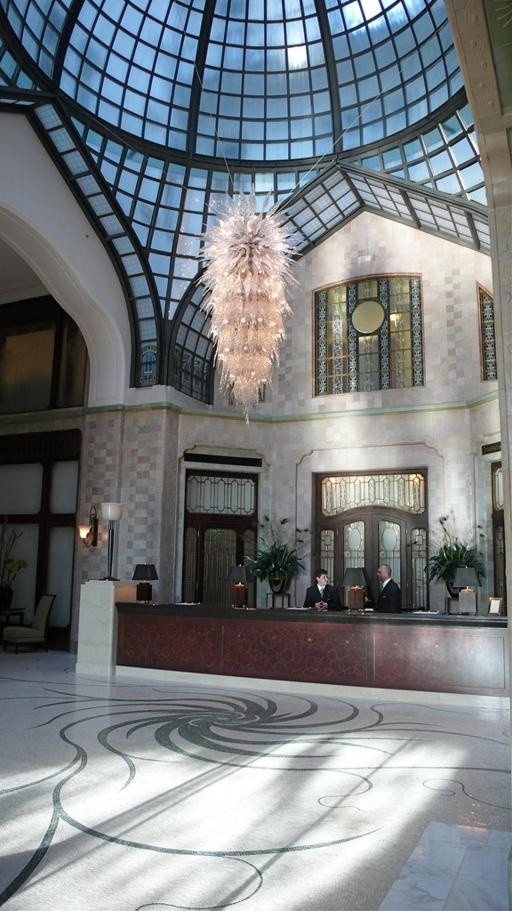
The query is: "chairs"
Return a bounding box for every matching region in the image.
[0,593,57,655]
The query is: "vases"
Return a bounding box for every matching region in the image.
[1,586,14,613]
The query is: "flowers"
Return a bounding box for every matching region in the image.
[0,517,30,589]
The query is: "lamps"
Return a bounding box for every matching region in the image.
[227,564,251,610]
[342,564,368,612]
[75,502,100,552]
[104,0,422,431]
[453,566,483,614]
[100,500,122,581]
[132,562,158,607]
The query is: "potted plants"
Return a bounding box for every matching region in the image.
[410,512,486,597]
[244,510,316,594]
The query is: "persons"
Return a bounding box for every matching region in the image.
[303,569,341,612]
[376,565,402,613]
